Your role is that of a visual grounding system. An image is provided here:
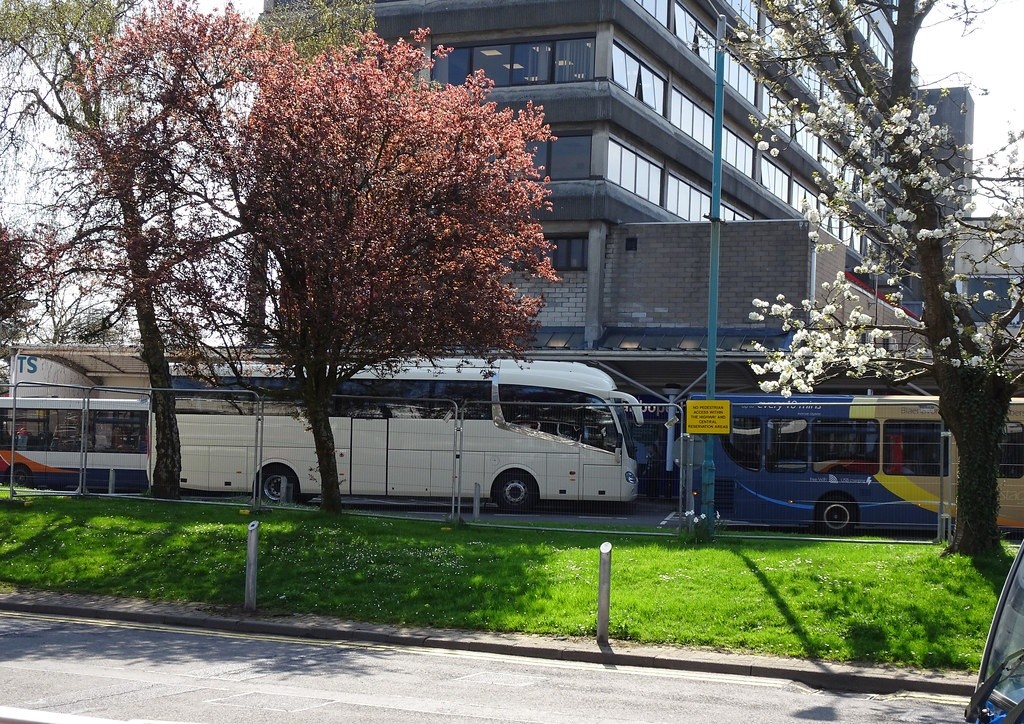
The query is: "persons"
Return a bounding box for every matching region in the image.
[643,443,662,503]
[4,427,146,454]
[740,438,920,477]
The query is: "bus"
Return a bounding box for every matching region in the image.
[145,353,644,510]
[0,397,148,491]
[686,391,1023,534]
